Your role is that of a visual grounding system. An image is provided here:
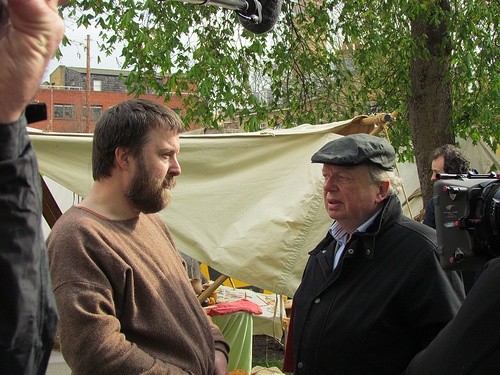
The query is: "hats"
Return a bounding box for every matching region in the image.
[310,133,395,171]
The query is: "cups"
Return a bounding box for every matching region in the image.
[192,278,202,293]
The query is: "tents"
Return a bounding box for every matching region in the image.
[25,114,426,299]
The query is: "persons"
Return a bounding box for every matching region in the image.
[404,256,500,375]
[288,132,467,375]
[44,96,232,375]
[0,0,68,374]
[419,143,471,233]
[179,249,204,297]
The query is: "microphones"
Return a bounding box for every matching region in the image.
[159,0,282,34]
